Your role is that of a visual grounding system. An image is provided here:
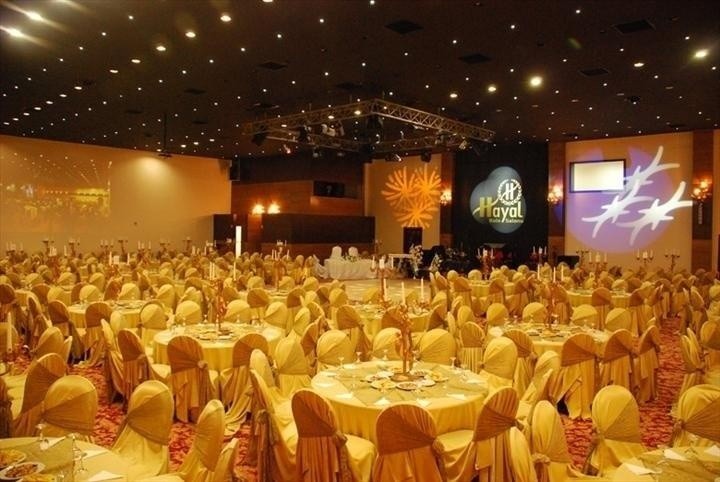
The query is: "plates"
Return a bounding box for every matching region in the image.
[370,383,396,389]
[390,368,409,374]
[365,375,388,381]
[410,369,432,377]
[201,333,214,340]
[2,462,45,480]
[423,375,448,381]
[420,379,435,386]
[557,332,570,337]
[2,448,26,468]
[538,334,554,338]
[376,371,394,377]
[397,382,422,390]
[218,335,231,340]
[537,327,544,331]
[528,330,540,335]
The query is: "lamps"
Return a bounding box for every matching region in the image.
[393,154,402,162]
[458,139,468,151]
[410,125,426,135]
[281,143,292,155]
[421,151,431,162]
[312,152,324,159]
[250,125,270,147]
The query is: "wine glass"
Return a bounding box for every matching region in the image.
[450,353,456,369]
[183,316,186,325]
[513,311,518,323]
[412,345,418,361]
[503,311,509,323]
[171,325,175,332]
[350,376,360,397]
[68,429,79,450]
[583,317,587,329]
[356,348,362,365]
[336,351,344,369]
[461,372,468,393]
[461,361,467,373]
[529,312,534,322]
[203,314,208,323]
[34,414,46,441]
[382,346,389,361]
[554,314,559,323]
[569,315,574,326]
[237,313,240,323]
[591,321,595,330]
